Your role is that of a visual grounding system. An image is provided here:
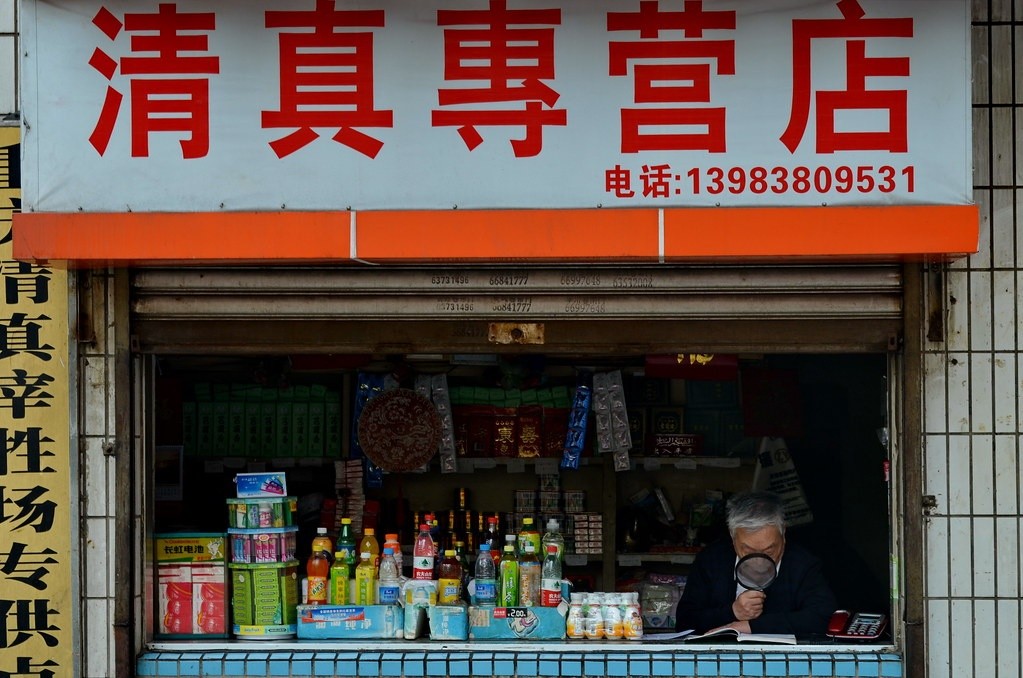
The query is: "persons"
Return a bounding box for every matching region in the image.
[675,489,837,635]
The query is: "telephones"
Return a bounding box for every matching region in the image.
[826,610,888,639]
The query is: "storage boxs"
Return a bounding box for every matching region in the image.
[156,496,567,638]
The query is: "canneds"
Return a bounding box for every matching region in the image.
[500,468,585,536]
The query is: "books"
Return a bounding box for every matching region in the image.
[684,628,812,645]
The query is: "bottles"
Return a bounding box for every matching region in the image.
[474,518,564,607]
[307,518,402,606]
[413,515,440,580]
[438,541,464,606]
[567,591,643,640]
[259,508,272,528]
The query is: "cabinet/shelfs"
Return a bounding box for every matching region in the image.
[202,353,764,592]
